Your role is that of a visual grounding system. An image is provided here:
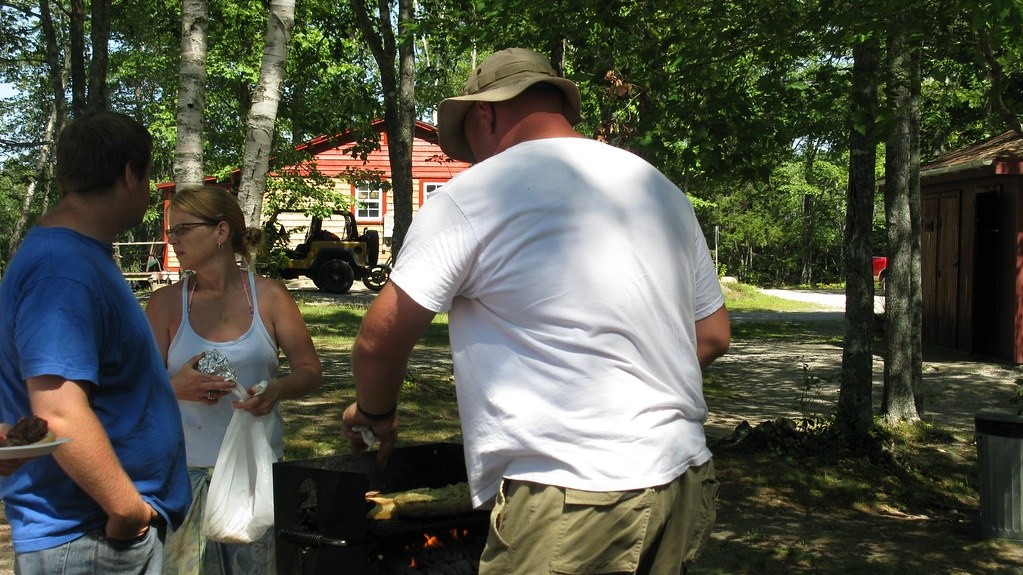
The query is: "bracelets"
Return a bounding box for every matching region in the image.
[357,402,396,421]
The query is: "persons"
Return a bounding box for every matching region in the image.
[0,111,193,575]
[144,186,322,575]
[341,48,730,575]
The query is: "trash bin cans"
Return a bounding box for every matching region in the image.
[973,405,1023,542]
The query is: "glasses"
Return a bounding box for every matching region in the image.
[165,222,216,239]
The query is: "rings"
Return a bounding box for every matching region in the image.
[208,391,210,399]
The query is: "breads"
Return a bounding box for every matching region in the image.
[30,429,57,444]
[394,481,472,515]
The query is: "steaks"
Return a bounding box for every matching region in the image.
[0,437,29,447]
[5,414,49,443]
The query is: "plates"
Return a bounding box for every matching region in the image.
[0,438,71,459]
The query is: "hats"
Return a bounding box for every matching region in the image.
[438,47,580,163]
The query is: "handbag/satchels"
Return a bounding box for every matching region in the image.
[200,376,282,542]
